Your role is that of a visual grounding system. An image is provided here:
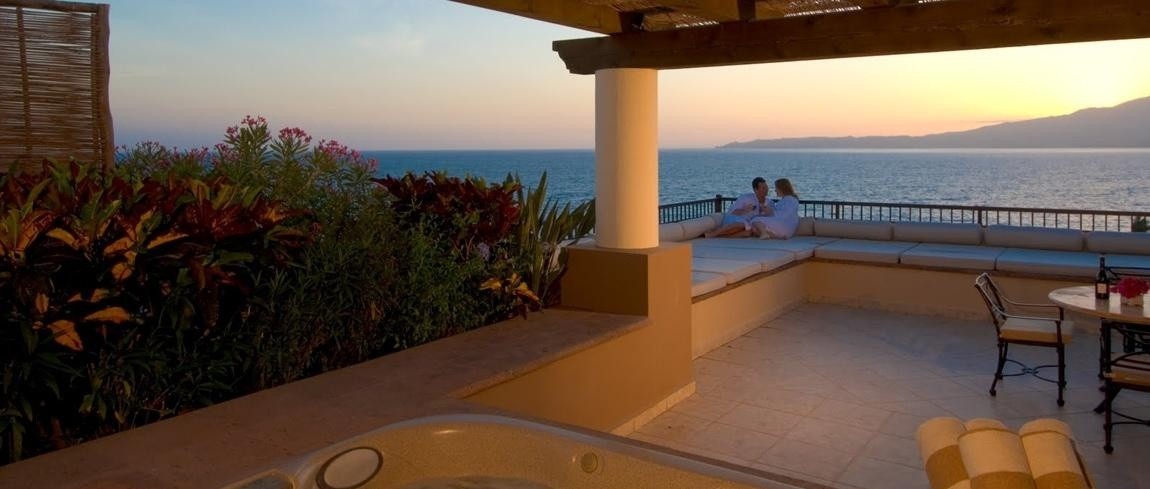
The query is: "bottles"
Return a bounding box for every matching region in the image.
[1095,256,1110,301]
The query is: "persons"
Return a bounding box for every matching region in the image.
[700,175,772,239]
[751,178,801,241]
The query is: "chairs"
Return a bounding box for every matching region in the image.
[972,265,1150,455]
